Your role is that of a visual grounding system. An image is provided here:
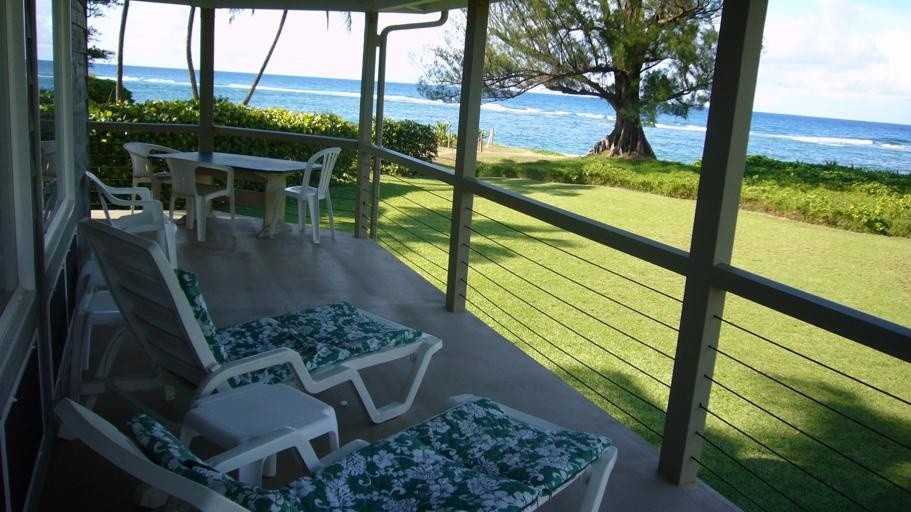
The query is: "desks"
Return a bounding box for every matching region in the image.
[146,149,325,240]
[67,288,131,384]
[178,381,341,487]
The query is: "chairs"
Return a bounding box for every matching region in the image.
[122,140,181,218]
[268,145,343,246]
[162,154,241,244]
[83,169,183,272]
[81,215,447,426]
[51,391,620,510]
